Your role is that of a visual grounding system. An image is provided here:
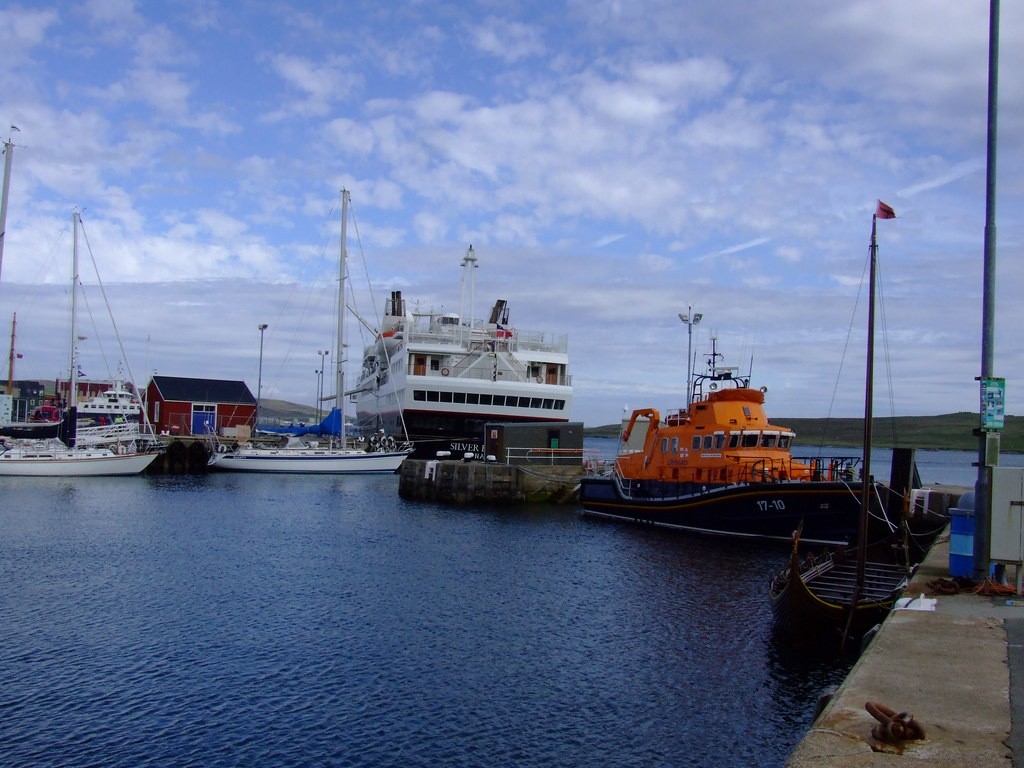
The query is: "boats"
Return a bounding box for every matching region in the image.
[576,301,877,537]
[345,243,575,453]
[78,378,142,426]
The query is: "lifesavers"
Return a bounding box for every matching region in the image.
[380,436,388,446]
[118,446,126,456]
[109,444,117,451]
[388,436,394,447]
[584,459,599,472]
[536,376,543,384]
[218,444,226,452]
[442,368,449,377]
[370,436,380,448]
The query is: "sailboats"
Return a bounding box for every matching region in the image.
[206,187,417,473]
[0,205,165,478]
[768,212,955,630]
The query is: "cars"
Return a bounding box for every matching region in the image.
[30,405,63,424]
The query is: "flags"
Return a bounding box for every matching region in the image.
[875,199,897,220]
[78,371,86,377]
[496,324,513,338]
[17,354,22,358]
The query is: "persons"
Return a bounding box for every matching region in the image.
[115,415,123,432]
[34,411,45,420]
[368,432,397,452]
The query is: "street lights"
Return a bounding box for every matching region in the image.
[318,349,331,425]
[315,370,324,429]
[256,322,268,438]
